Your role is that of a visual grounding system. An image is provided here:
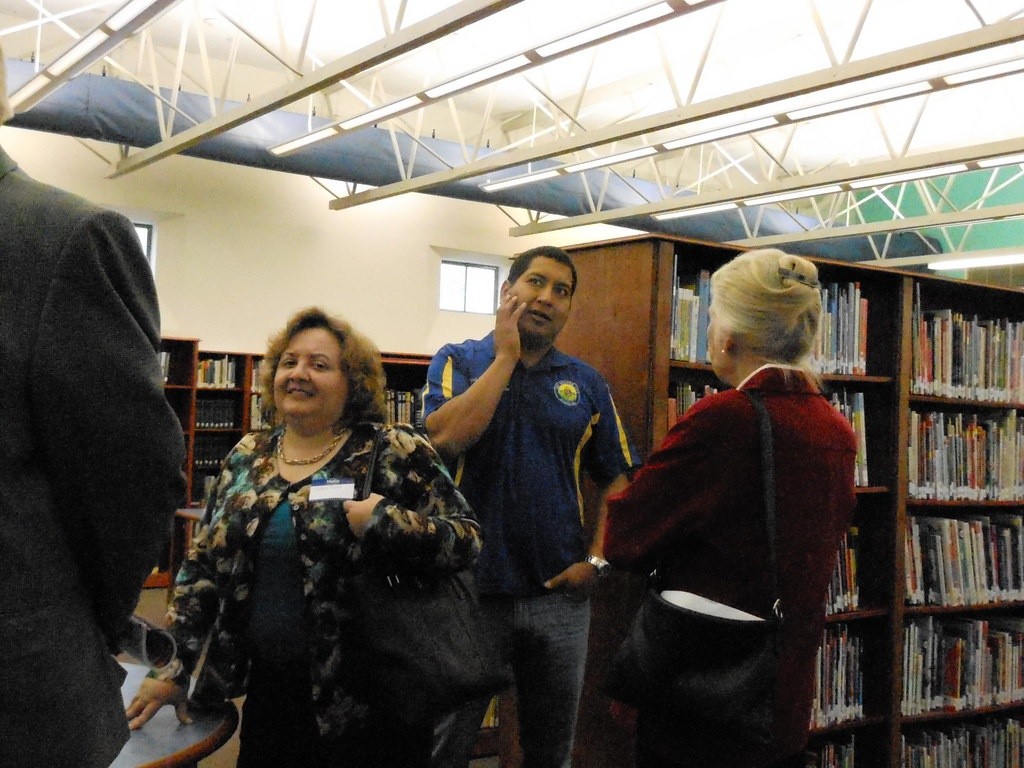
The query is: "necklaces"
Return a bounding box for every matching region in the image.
[277,425,347,464]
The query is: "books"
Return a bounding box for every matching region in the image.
[801,528,865,768]
[907,409,1024,502]
[904,515,1024,606]
[900,718,1024,768]
[668,381,869,487]
[672,252,867,377]
[902,614,1024,717]
[912,282,1024,404]
[158,347,423,502]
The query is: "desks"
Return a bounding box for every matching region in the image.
[110,662,241,768]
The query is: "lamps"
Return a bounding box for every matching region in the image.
[7,0,1024,270]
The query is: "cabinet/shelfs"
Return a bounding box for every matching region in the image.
[514,232,1023,768]
[144,338,201,589]
[191,349,436,548]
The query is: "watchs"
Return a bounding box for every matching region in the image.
[586,555,611,578]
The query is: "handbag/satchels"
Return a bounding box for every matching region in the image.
[598,392,790,746]
[353,426,522,734]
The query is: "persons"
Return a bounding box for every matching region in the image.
[602,247,855,768]
[123,308,486,767]
[419,246,641,768]
[0,144,186,768]
